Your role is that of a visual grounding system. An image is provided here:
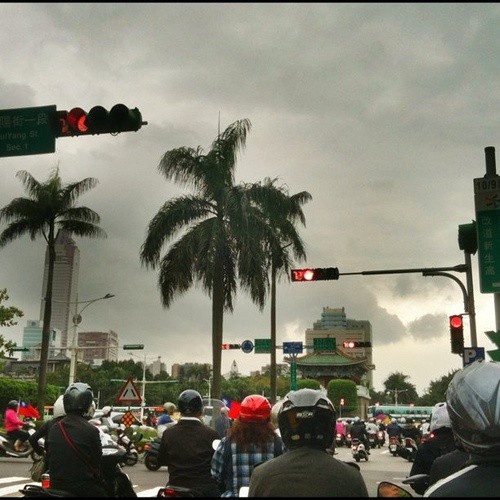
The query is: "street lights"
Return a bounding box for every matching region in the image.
[127,352,161,425]
[41,292,115,384]
[203,377,214,405]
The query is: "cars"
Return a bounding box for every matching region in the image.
[107,411,155,432]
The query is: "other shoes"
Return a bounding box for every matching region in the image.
[366,450,371,455]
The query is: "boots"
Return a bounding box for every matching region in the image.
[14,439,27,452]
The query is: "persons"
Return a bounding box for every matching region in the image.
[410,361,500,500]
[156,401,176,426]
[83,401,126,465]
[158,390,222,498]
[249,388,369,499]
[337,416,430,456]
[29,382,116,500]
[210,394,285,497]
[217,407,232,435]
[5,400,29,451]
[378,482,401,497]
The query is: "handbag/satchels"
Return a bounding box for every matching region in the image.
[95,471,114,497]
[28,450,49,482]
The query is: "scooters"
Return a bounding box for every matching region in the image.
[0,427,137,499]
[0,420,47,461]
[142,436,168,470]
[337,427,432,463]
[109,421,139,467]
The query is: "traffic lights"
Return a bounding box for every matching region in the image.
[344,342,371,348]
[221,343,242,350]
[449,315,465,353]
[290,266,340,281]
[57,104,143,137]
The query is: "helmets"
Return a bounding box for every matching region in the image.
[336,417,352,423]
[271,399,287,426]
[102,405,113,415]
[220,407,230,414]
[163,402,176,415]
[8,400,19,407]
[354,416,383,424]
[430,402,453,432]
[392,416,430,423]
[63,382,94,413]
[277,389,338,450]
[443,360,500,456]
[53,394,67,419]
[82,400,96,419]
[178,389,203,414]
[240,394,272,421]
